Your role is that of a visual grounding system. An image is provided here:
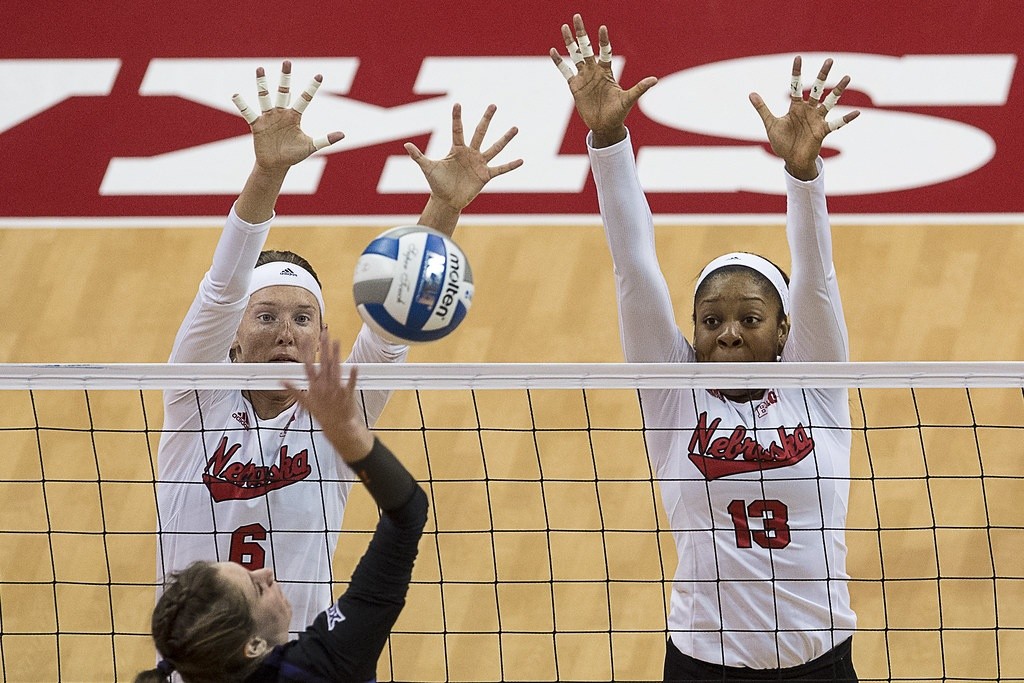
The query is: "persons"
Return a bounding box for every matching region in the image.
[132,328,429,683]
[549,15,861,683]
[154,62,527,683]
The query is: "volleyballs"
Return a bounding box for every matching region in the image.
[353,224,475,344]
